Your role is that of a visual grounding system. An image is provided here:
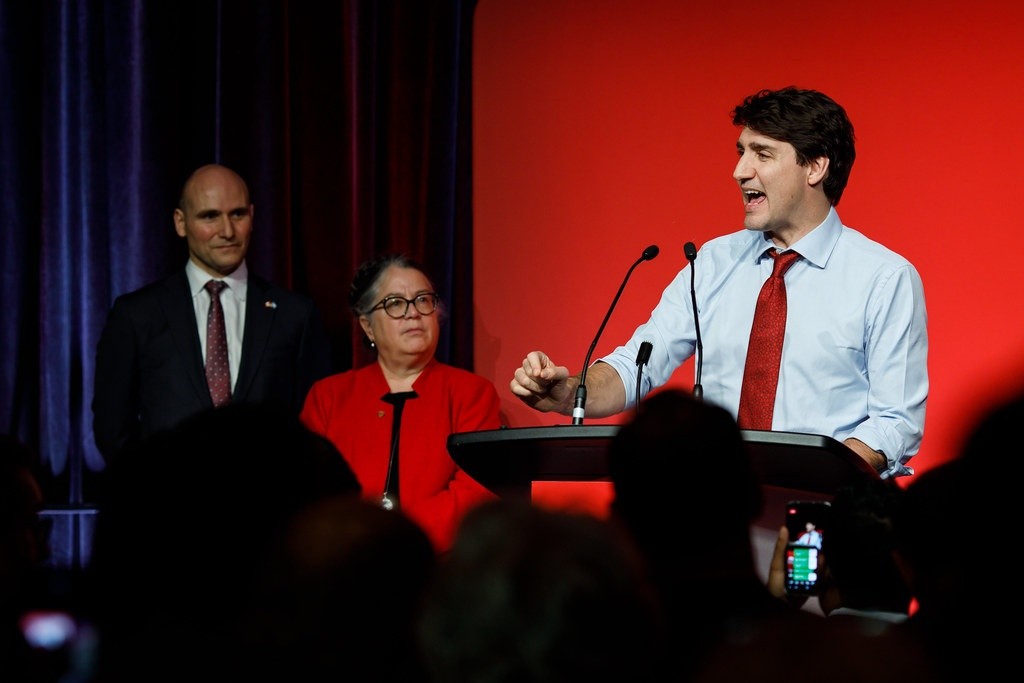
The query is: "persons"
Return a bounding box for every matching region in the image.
[790,522,822,550]
[0,389,1024,683]
[91,163,320,464]
[509,85,928,483]
[298,250,501,556]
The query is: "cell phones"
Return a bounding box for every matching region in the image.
[783,500,836,607]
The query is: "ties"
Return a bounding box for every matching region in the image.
[203,280,232,405]
[736,250,801,431]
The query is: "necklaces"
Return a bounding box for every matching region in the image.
[381,415,400,512]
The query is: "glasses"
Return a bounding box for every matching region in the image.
[366,293,440,319]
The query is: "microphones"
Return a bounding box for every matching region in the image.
[635,341,653,413]
[573,245,659,425]
[684,242,704,399]
